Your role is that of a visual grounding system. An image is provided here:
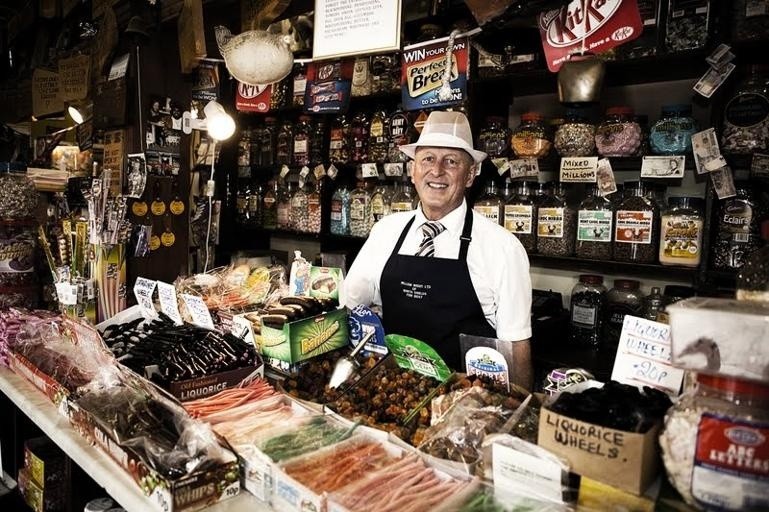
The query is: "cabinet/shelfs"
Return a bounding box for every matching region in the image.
[469,44,724,284]
[323,51,469,255]
[221,60,322,244]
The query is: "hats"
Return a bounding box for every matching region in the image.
[398,110,489,165]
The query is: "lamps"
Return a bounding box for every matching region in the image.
[180,100,236,142]
[28,96,95,140]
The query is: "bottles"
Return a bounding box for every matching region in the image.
[331,182,347,235]
[330,115,348,164]
[504,181,533,248]
[348,182,369,237]
[601,280,646,362]
[369,185,389,228]
[350,109,370,164]
[656,196,704,272]
[231,120,290,167]
[293,121,308,162]
[568,274,606,350]
[371,109,389,160]
[237,182,289,229]
[292,182,306,231]
[574,185,615,254]
[533,185,569,260]
[391,183,412,214]
[306,183,322,233]
[387,109,410,162]
[611,178,658,261]
[473,179,501,225]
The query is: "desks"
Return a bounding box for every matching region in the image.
[0,360,272,512]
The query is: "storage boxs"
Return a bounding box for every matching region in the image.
[66,394,239,512]
[232,413,368,502]
[326,454,462,512]
[273,433,415,512]
[7,344,70,407]
[575,476,662,512]
[150,356,265,402]
[261,305,350,365]
[538,391,669,497]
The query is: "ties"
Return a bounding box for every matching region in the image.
[414,221,446,259]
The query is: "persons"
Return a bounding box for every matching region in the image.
[339,110,534,393]
[697,153,715,172]
[667,159,680,175]
